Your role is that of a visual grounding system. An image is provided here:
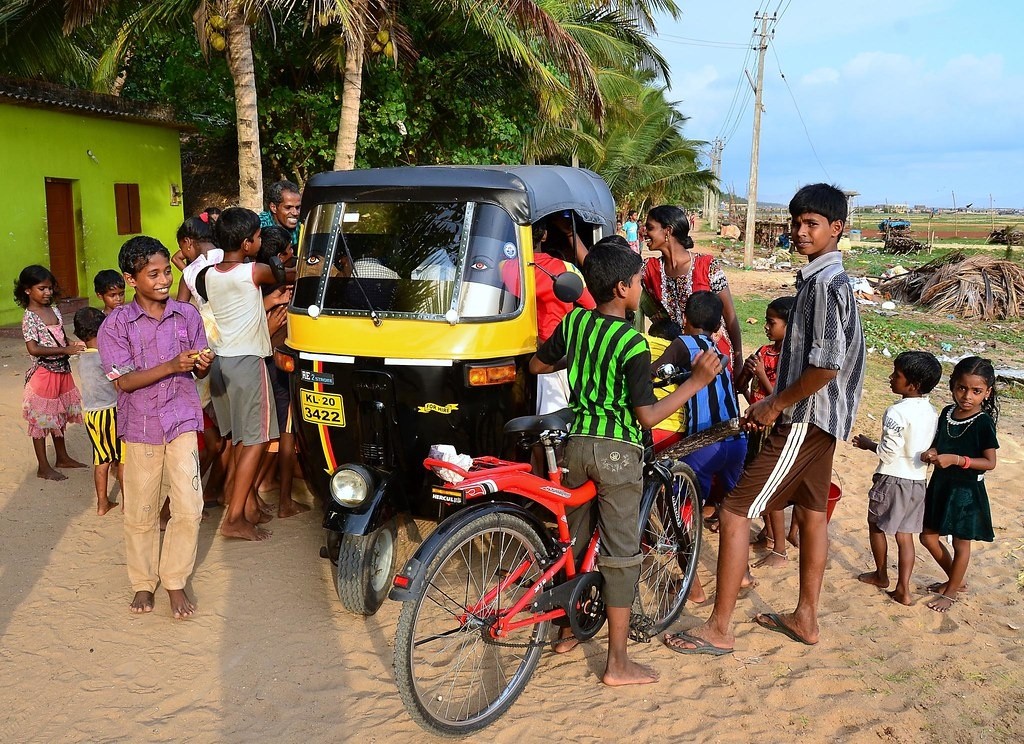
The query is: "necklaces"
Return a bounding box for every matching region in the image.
[221,260,240,263]
[947,421,973,439]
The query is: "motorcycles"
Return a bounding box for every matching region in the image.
[269,166,622,613]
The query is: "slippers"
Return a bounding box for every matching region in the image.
[756,613,815,645]
[664,629,735,655]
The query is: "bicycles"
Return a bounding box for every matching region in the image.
[388,360,725,740]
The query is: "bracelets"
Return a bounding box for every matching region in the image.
[567,232,573,237]
[956,455,960,465]
[962,456,970,469]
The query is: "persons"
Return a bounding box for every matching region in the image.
[920,356,1001,613]
[97,236,215,618]
[498,182,866,683]
[851,352,943,605]
[74,180,458,541]
[13,265,89,481]
